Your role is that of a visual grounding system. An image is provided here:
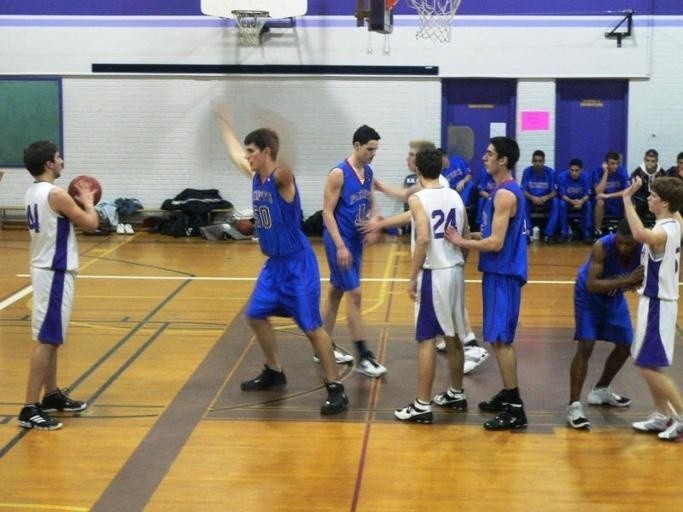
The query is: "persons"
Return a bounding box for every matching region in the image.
[623,174,683,441]
[312,124,417,376]
[394,149,470,424]
[16,140,99,431]
[568,217,644,428]
[446,136,528,431]
[357,141,490,377]
[217,105,349,414]
[436,148,683,244]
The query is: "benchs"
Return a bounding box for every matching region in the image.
[529,212,658,240]
[0,206,231,231]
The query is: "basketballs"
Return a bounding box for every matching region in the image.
[237,220,253,235]
[68,175,102,210]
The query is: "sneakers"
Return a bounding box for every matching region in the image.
[478,388,529,432]
[527,231,603,245]
[239,364,288,390]
[566,399,591,429]
[318,377,350,415]
[631,412,682,441]
[393,397,435,425]
[352,350,390,377]
[588,382,632,407]
[116,221,136,236]
[40,386,90,413]
[432,385,469,411]
[17,402,63,433]
[462,335,488,374]
[312,343,355,365]
